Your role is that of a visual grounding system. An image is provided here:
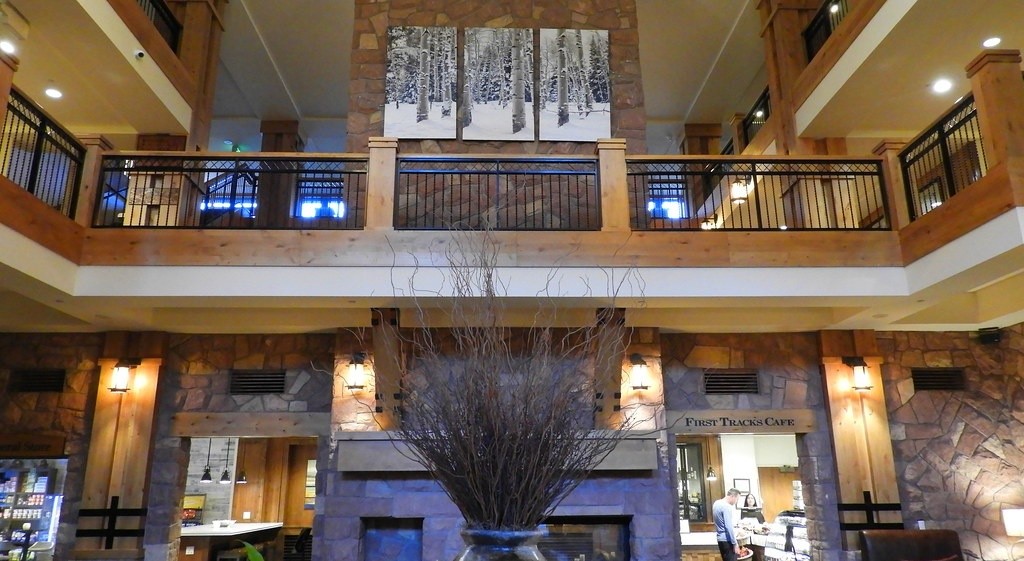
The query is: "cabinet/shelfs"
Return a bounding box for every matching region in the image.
[0,467,58,551]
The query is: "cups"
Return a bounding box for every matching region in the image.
[0,466,50,518]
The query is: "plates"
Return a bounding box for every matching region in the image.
[211,519,237,528]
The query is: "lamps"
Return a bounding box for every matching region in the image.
[841,357,874,391]
[344,352,367,389]
[200,438,213,483]
[629,353,652,391]
[978,326,1002,344]
[106,358,141,394]
[730,173,751,205]
[705,434,717,481]
[235,439,247,484]
[220,437,231,484]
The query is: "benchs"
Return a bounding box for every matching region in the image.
[215,540,277,561]
[859,530,965,561]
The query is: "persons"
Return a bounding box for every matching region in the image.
[741,493,765,524]
[713,488,741,561]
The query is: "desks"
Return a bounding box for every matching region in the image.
[177,522,283,561]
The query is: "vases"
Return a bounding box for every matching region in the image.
[452,529,547,561]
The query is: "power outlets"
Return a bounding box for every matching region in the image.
[185,546,195,556]
